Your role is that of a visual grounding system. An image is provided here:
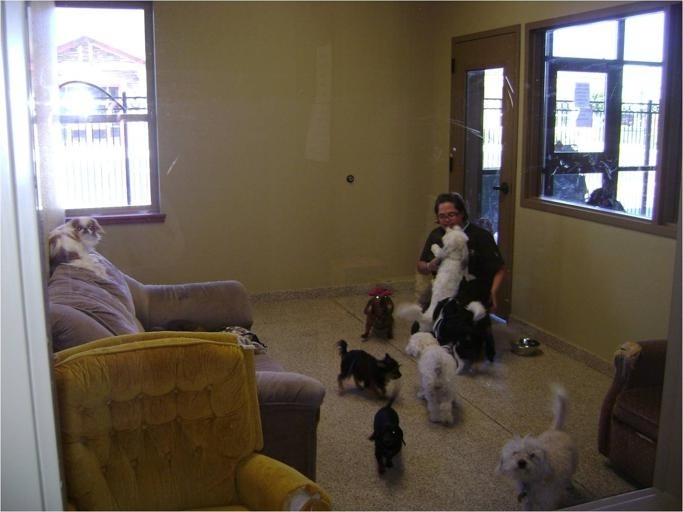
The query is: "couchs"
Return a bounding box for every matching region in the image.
[45,249,327,483]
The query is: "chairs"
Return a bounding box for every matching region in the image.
[597,339,667,489]
[48,332,335,510]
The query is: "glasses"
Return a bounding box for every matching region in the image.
[438,212,456,221]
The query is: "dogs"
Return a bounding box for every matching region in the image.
[361,295,393,342]
[367,388,407,467]
[48,215,116,279]
[335,339,402,399]
[405,329,457,427]
[432,296,496,375]
[392,225,475,332]
[494,381,576,510]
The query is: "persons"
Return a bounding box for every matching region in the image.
[409,192,507,363]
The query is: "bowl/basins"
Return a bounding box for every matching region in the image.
[507,336,541,357]
